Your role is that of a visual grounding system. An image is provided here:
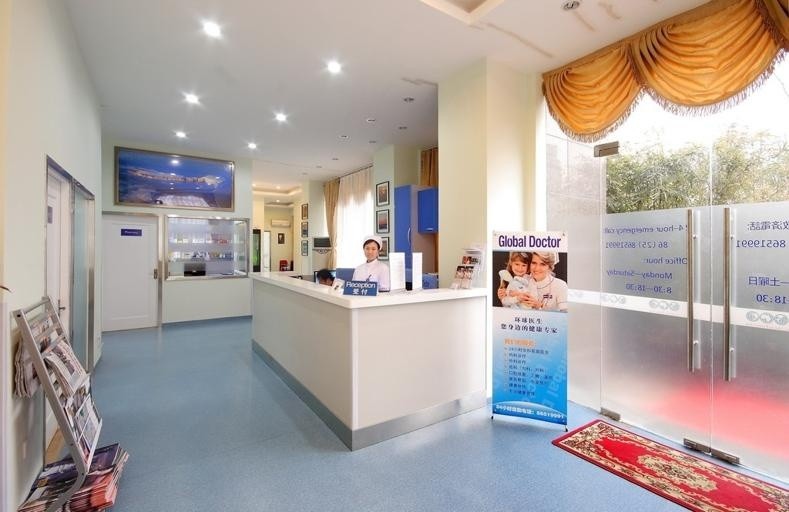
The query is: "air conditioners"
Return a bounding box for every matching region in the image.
[271,220,290,228]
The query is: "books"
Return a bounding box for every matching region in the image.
[17,442,130,512]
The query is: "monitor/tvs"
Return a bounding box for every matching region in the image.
[184,263,205,276]
[314,270,336,284]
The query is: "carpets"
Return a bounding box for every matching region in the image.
[549,418,789,512]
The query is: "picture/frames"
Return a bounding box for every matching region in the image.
[301,203,308,256]
[113,143,238,213]
[375,180,390,262]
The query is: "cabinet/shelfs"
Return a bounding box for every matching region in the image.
[163,214,250,281]
[395,184,438,283]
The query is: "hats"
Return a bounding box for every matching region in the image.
[362,237,382,250]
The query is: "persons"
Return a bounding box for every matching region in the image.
[498,252,567,311]
[317,269,345,289]
[350,236,389,291]
